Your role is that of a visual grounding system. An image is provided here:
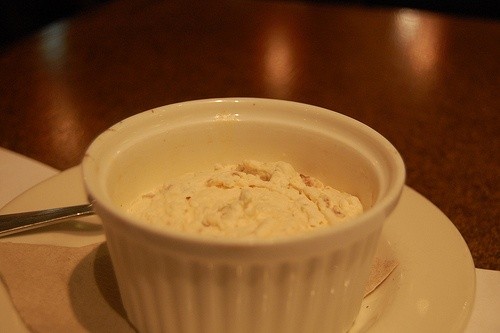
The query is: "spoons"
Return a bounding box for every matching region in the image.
[1,200,101,241]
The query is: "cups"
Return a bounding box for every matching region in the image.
[79,96,408,332]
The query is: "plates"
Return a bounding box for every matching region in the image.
[2,157,479,332]
